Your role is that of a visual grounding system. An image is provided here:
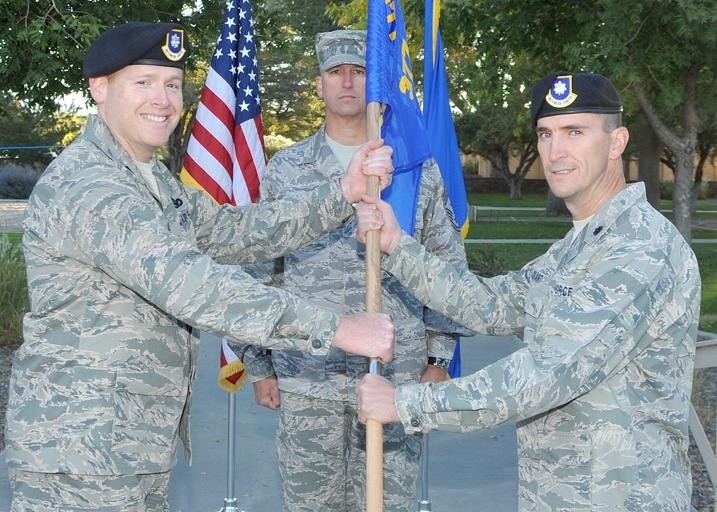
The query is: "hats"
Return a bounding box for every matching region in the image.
[314,28,367,73]
[83,20,191,78]
[530,70,624,132]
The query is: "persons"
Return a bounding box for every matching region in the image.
[356,70,701,511]
[4,22,395,512]
[227,29,468,512]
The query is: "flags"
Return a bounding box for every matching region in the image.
[180,0,268,394]
[366,0,468,381]
